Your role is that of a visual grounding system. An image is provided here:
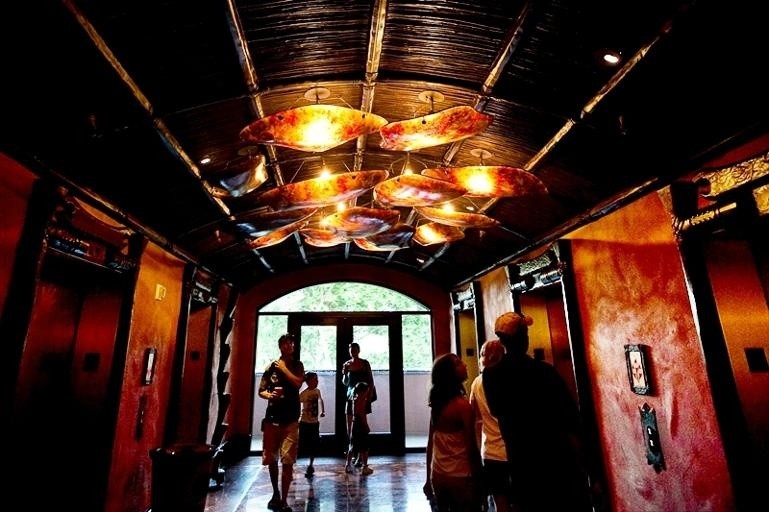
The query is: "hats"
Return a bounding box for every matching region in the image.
[279,334,296,348]
[495,312,533,336]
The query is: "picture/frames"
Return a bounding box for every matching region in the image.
[623,343,654,395]
[140,347,157,387]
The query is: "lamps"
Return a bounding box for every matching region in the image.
[380,95,494,152]
[240,91,389,152]
[205,150,547,251]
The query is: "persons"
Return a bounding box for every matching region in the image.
[300,372,326,474]
[344,383,373,474]
[482,310,584,511]
[258,335,305,511]
[422,352,484,512]
[469,340,523,512]
[343,342,377,465]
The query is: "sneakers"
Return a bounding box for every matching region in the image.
[268,492,280,509]
[363,468,373,475]
[354,460,362,467]
[345,465,353,473]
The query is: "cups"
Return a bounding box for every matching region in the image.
[274,386,283,398]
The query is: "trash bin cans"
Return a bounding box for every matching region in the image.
[149,448,212,512]
